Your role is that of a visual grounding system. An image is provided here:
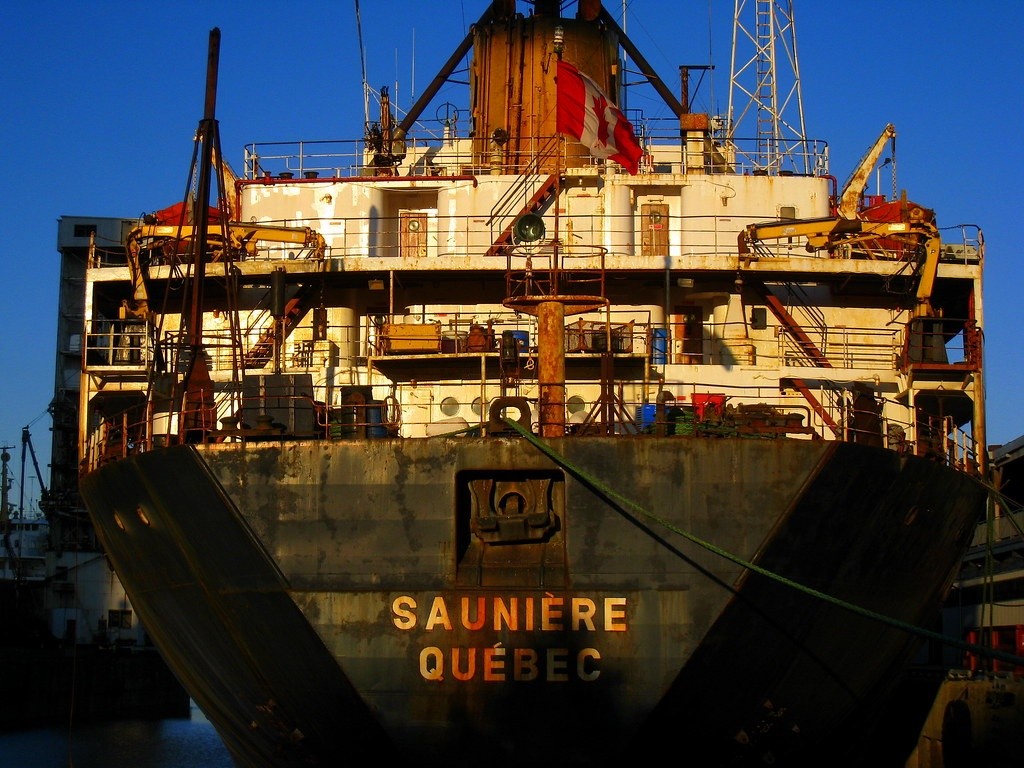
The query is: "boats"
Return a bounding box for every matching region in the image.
[935,436,1024,768]
[0,213,193,736]
[77,0,988,768]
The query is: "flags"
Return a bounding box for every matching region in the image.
[556,59,643,176]
